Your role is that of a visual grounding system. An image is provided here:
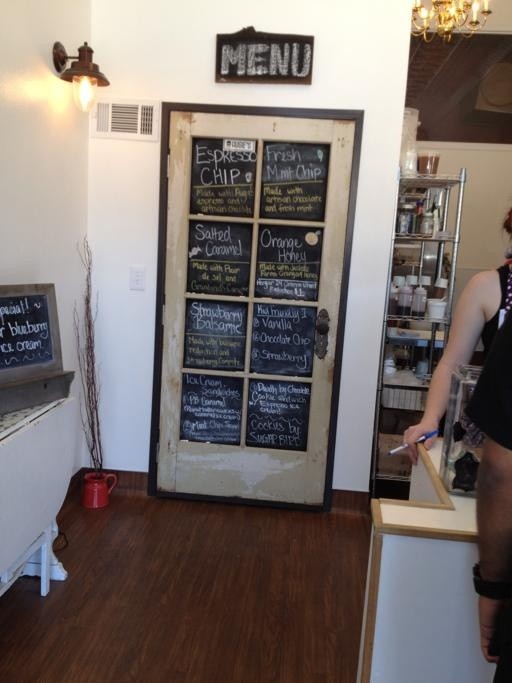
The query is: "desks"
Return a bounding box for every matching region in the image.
[0,397,75,596]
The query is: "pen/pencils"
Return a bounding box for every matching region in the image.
[387,429,438,456]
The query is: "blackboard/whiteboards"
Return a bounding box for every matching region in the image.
[189,136,258,218]
[245,376,311,454]
[179,369,244,448]
[187,218,254,298]
[250,301,318,379]
[182,296,248,373]
[214,32,315,85]
[259,141,331,221]
[253,224,325,303]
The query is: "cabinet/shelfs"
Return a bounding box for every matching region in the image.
[370,166,467,497]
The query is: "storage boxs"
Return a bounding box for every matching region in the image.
[378,409,441,477]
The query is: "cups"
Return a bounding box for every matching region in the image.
[417,149,442,177]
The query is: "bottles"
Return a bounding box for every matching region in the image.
[382,338,444,376]
[396,198,435,238]
[392,275,449,322]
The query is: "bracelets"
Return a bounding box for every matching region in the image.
[470,564,508,601]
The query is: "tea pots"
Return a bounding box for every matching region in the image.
[81,472,118,509]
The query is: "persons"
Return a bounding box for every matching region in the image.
[402,209,512,466]
[462,310,512,683]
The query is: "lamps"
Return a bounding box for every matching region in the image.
[411,0,492,43]
[53,41,109,113]
